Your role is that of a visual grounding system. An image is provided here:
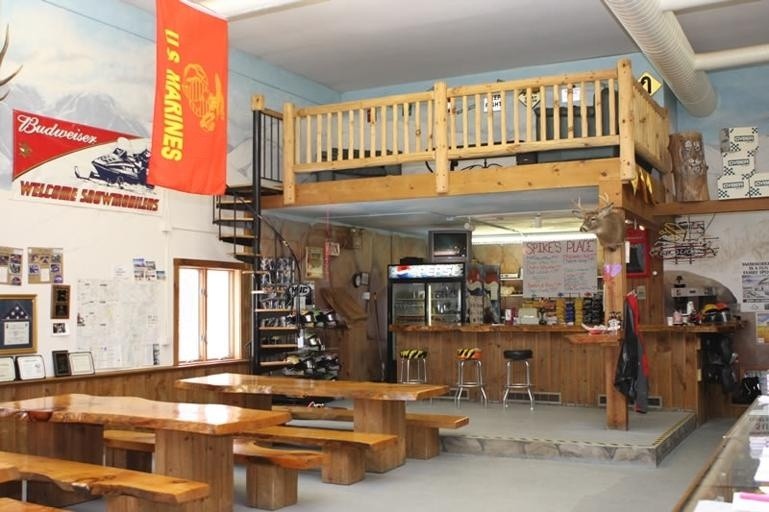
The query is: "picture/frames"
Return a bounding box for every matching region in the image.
[0,293,38,355]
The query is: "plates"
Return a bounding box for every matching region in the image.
[588,330,603,334]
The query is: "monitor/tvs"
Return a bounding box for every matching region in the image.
[428,230,471,263]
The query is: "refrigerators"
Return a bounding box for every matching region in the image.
[387,262,466,382]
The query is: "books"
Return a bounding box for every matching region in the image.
[731,491,769,511]
[748,414,769,461]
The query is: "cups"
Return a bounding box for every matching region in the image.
[667,317,674,326]
[681,313,689,326]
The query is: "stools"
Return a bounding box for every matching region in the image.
[454,348,488,408]
[502,349,536,411]
[399,349,427,405]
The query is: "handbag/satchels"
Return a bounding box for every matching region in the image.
[742,377,761,404]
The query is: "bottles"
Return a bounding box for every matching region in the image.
[505,309,512,325]
[611,319,617,335]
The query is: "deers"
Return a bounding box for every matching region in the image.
[572,192,625,249]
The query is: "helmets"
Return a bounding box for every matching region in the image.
[298,330,325,352]
[701,303,733,323]
[282,353,342,380]
[299,307,338,328]
